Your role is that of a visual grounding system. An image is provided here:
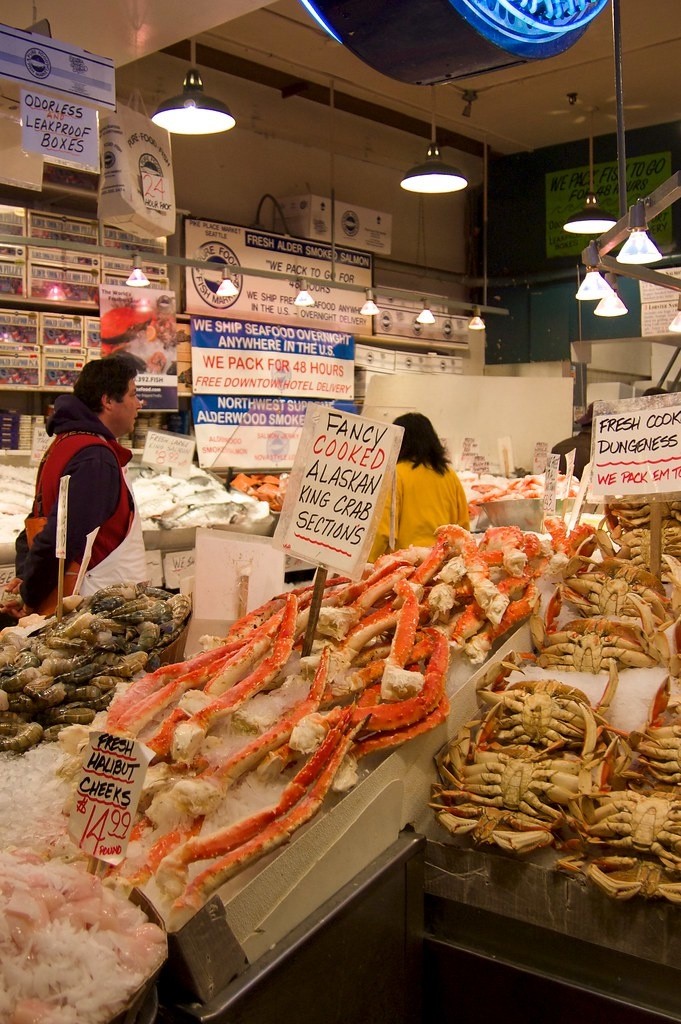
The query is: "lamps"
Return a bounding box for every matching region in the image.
[575,239,614,301]
[594,272,629,317]
[561,104,617,234]
[216,265,240,296]
[360,288,380,315]
[469,305,485,330]
[615,199,663,265]
[417,297,435,324]
[669,294,681,332]
[294,277,315,306]
[399,85,468,194]
[124,251,151,287]
[151,34,236,136]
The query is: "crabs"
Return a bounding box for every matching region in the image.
[64,492,681,917]
[100,307,158,353]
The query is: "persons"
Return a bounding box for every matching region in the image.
[368,413,468,563]
[0,356,148,618]
[552,399,605,480]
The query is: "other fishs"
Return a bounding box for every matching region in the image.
[129,467,250,531]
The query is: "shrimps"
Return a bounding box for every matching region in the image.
[0,579,191,757]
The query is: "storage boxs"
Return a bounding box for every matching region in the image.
[431,355,464,372]
[353,366,395,397]
[0,204,169,305]
[40,313,83,349]
[177,324,193,354]
[84,317,101,349]
[42,348,85,386]
[375,306,412,343]
[0,344,41,386]
[177,362,192,392]
[273,195,394,255]
[0,309,39,345]
[413,313,442,342]
[353,343,395,370]
[374,286,449,314]
[395,351,432,371]
[442,313,471,343]
